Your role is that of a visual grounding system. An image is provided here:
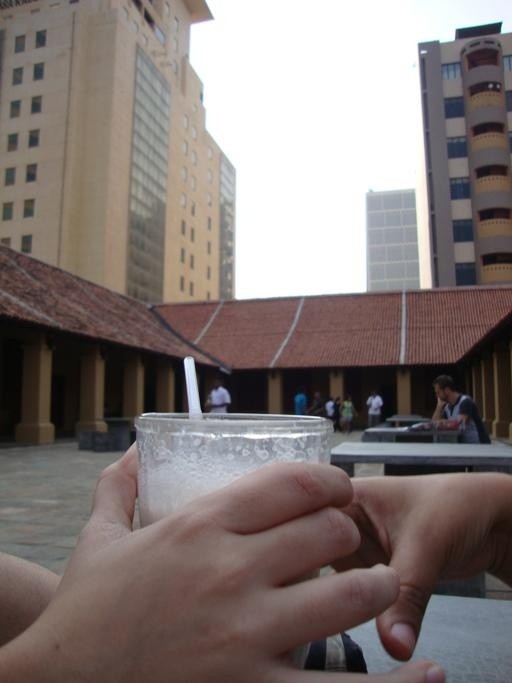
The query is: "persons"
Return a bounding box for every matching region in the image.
[204,379,231,413]
[0,441,446,682]
[294,385,384,434]
[324,470,512,662]
[408,374,492,444]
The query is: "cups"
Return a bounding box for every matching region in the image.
[135,408,334,538]
